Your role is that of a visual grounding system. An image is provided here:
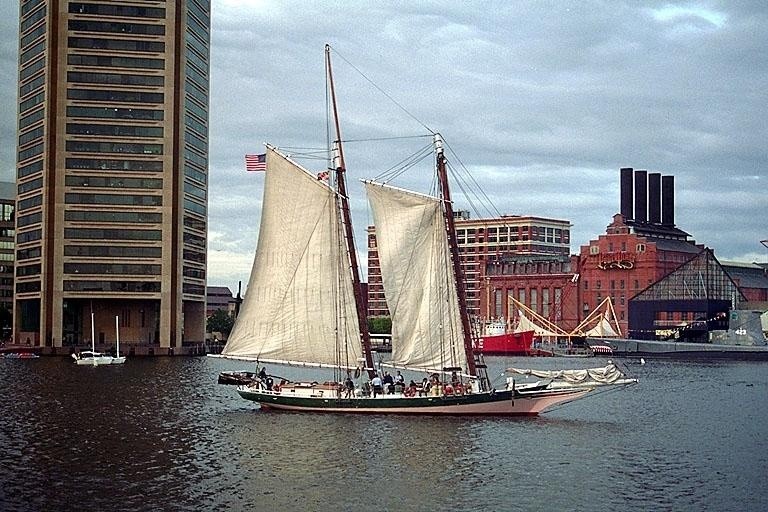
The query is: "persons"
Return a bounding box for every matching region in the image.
[258,365,267,380]
[345,370,473,398]
[266,374,274,394]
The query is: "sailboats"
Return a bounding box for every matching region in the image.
[72,311,125,366]
[237,42,644,417]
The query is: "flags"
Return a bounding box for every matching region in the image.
[317,172,329,180]
[245,154,265,171]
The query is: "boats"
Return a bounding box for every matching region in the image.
[471,314,536,355]
[579,310,768,364]
[3,352,40,359]
[216,369,255,383]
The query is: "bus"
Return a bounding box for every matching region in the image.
[369,334,392,344]
